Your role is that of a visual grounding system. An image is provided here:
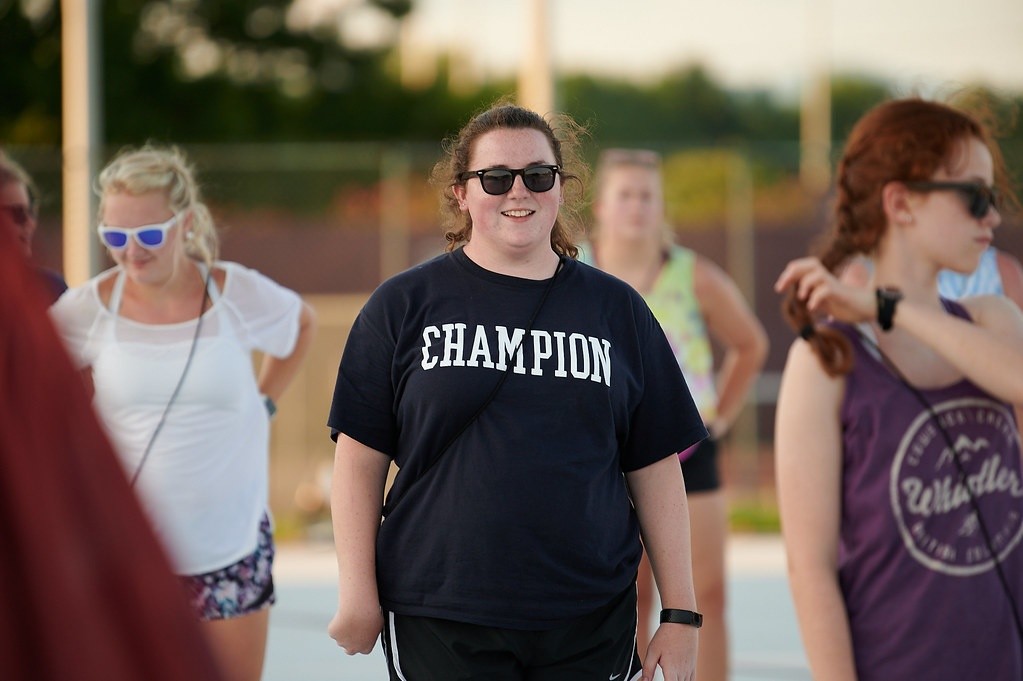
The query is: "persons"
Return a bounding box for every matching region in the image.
[577,148,769,681]
[1,153,68,310]
[828,247,1022,447]
[775,99,1023,680]
[46,150,316,680]
[321,106,708,680]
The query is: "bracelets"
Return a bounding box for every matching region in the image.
[660,608,703,629]
[876,284,902,331]
[257,393,277,415]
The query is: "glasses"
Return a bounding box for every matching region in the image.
[459,164,562,196]
[11,205,33,226]
[96,212,184,249]
[911,177,1001,218]
